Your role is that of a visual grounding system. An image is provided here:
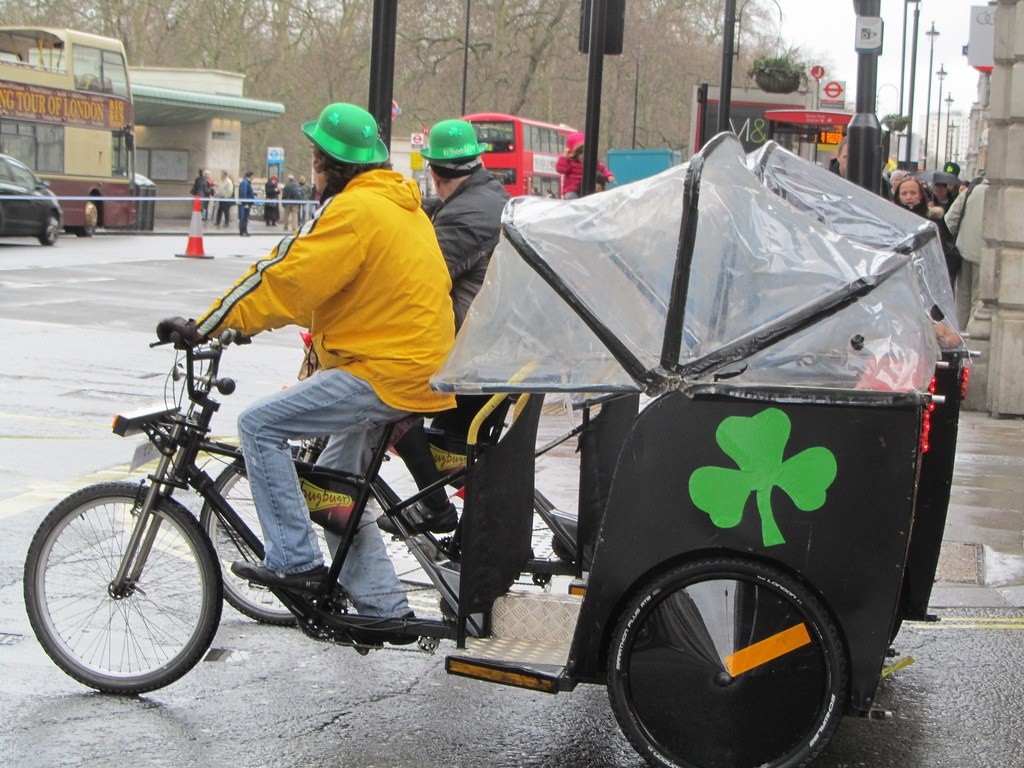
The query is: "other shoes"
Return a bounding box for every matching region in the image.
[377,501,458,535]
[334,611,419,645]
[231,561,330,599]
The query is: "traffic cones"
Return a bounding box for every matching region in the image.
[174,195,215,260]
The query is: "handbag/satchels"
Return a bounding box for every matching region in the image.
[948,247,964,271]
[190,178,199,194]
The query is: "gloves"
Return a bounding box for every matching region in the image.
[156,318,208,347]
[234,330,252,345]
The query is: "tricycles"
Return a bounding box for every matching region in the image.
[23,132,985,768]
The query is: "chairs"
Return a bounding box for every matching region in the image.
[73,74,114,95]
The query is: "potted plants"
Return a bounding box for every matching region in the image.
[880,113,912,134]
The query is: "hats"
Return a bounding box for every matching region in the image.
[301,103,390,163]
[567,133,584,157]
[944,162,960,177]
[419,119,489,162]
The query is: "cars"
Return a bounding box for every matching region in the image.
[0,154,65,247]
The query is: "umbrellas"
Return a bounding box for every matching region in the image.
[913,170,964,201]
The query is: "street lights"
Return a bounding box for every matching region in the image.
[943,91,955,163]
[935,63,948,168]
[923,21,941,169]
[948,120,957,162]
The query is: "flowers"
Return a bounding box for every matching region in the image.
[747,47,811,95]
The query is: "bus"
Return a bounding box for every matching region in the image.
[460,111,579,200]
[0,25,139,239]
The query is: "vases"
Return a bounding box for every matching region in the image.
[755,72,800,94]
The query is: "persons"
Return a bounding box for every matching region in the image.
[547,189,555,198]
[596,175,606,193]
[884,170,904,200]
[929,183,954,213]
[532,186,541,195]
[556,132,614,200]
[894,177,962,278]
[959,180,970,194]
[828,136,847,179]
[944,176,990,331]
[376,118,512,535]
[195,169,236,226]
[282,175,304,231]
[298,176,312,225]
[239,172,259,236]
[155,102,458,645]
[263,176,281,226]
